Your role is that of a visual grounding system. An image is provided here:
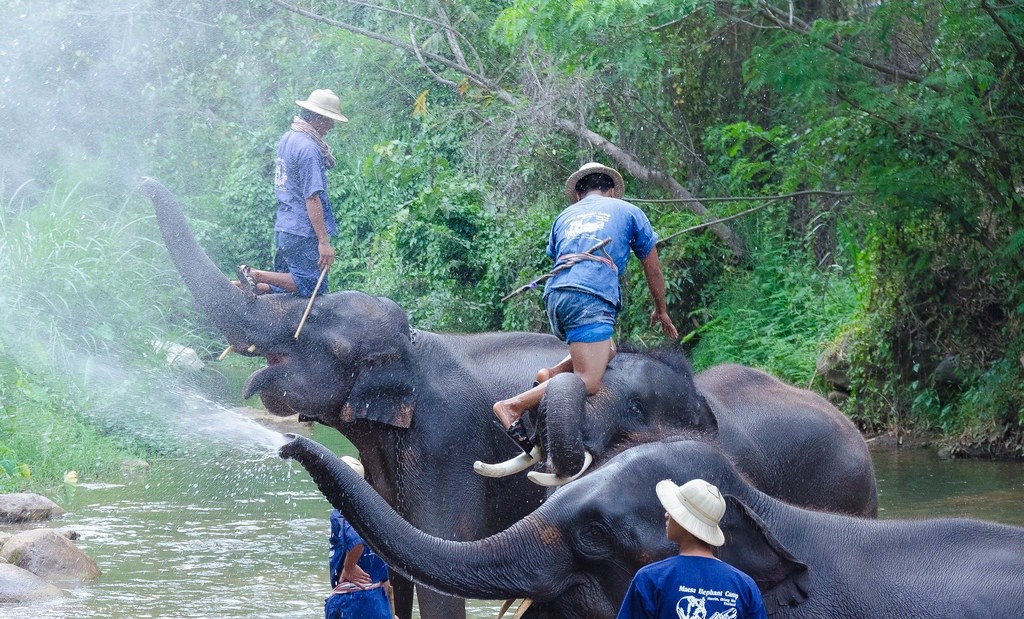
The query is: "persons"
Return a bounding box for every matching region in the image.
[324,456,399,619]
[616,478,768,619]
[230,88,348,301]
[490,162,679,460]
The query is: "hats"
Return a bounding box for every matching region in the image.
[295,88,349,123]
[565,162,625,205]
[655,479,726,547]
[341,455,366,481]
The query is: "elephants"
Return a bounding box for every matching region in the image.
[140,175,1024,619]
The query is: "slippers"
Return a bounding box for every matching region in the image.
[529,381,541,423]
[493,417,535,460]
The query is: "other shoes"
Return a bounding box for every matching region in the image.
[235,263,258,306]
